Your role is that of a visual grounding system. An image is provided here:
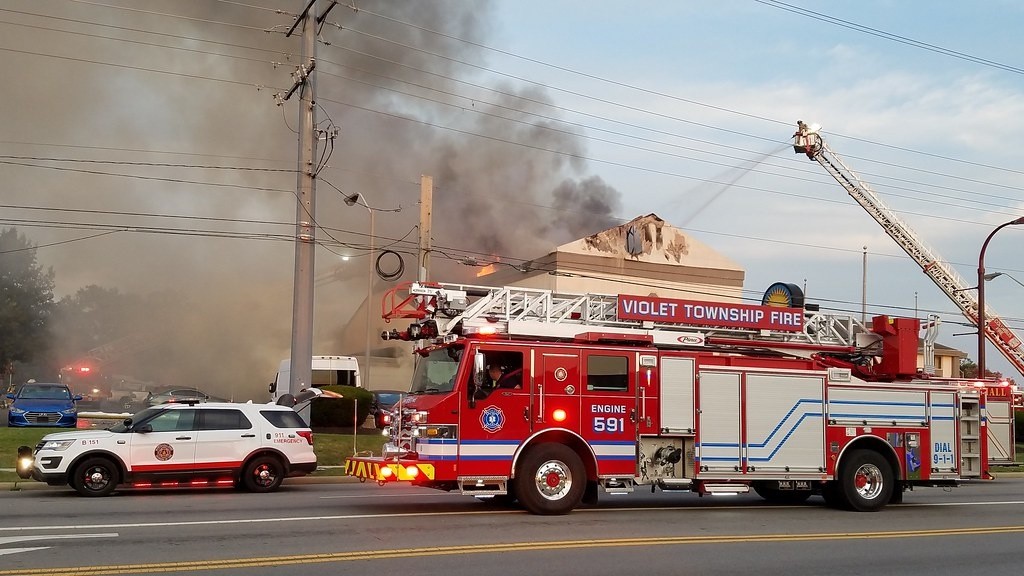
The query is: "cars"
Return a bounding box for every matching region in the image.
[144,386,231,409]
[369,390,408,428]
[7,383,83,429]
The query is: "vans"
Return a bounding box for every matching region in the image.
[269,356,360,408]
[106,379,162,409]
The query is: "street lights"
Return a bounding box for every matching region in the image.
[344,190,374,391]
[978,217,1024,378]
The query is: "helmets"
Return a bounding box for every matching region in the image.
[487,354,517,372]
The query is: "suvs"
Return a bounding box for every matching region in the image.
[15,387,343,496]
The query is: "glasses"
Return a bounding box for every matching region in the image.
[486,364,492,369]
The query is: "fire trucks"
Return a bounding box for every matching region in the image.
[794,130,1024,373]
[344,282,1024,518]
[59,262,367,407]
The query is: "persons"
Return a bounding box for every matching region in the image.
[488,363,521,389]
